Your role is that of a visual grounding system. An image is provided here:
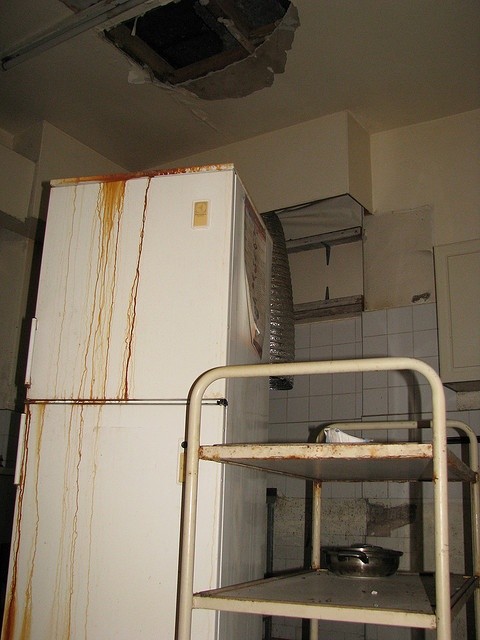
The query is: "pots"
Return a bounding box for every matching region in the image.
[324,545,404,579]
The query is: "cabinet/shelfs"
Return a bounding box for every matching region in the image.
[174,356,480,636]
[284,225,364,320]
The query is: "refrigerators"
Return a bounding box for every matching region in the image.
[0,164,272,640]
